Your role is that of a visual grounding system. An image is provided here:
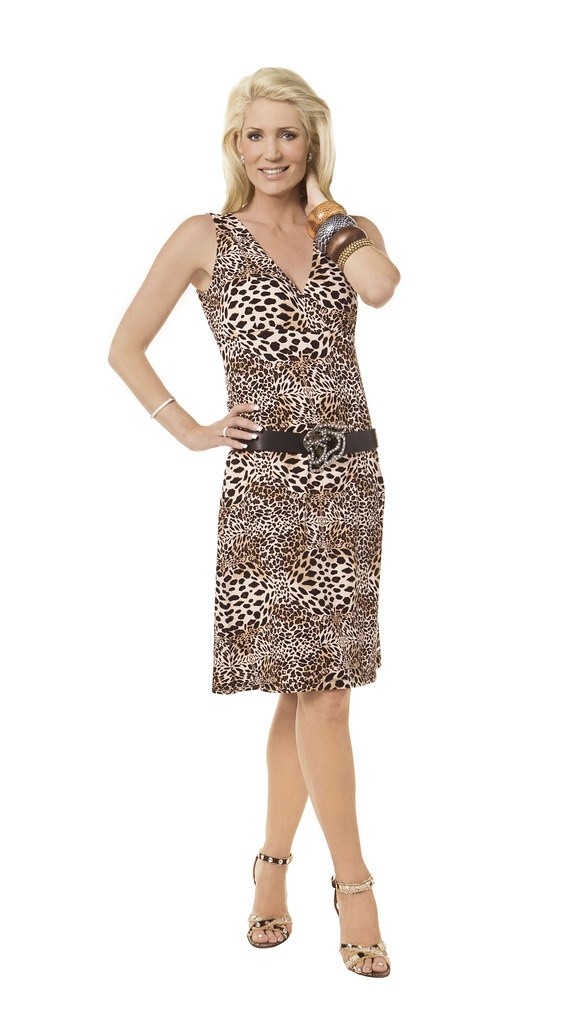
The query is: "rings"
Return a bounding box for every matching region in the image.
[223,427,228,437]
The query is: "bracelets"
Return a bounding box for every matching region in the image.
[337,238,372,271]
[305,200,347,240]
[151,397,176,418]
[315,214,357,254]
[327,226,367,262]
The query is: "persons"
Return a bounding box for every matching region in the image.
[107,68,400,979]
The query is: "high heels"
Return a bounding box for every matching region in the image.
[246,852,294,948]
[331,873,390,978]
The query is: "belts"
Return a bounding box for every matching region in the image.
[235,428,377,472]
